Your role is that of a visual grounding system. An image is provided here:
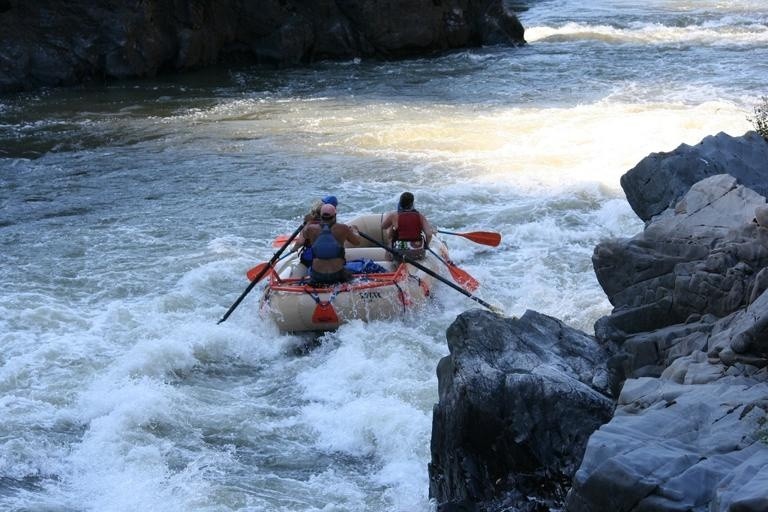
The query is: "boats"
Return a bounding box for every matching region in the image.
[259,213,448,336]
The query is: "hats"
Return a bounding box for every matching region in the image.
[320,205,338,218]
[321,195,338,207]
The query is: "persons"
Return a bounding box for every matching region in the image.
[387,201,438,242]
[381,191,433,265]
[304,195,338,222]
[291,200,324,267]
[303,203,361,285]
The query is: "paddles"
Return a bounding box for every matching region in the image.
[247,250,293,283]
[358,231,515,320]
[274,236,298,246]
